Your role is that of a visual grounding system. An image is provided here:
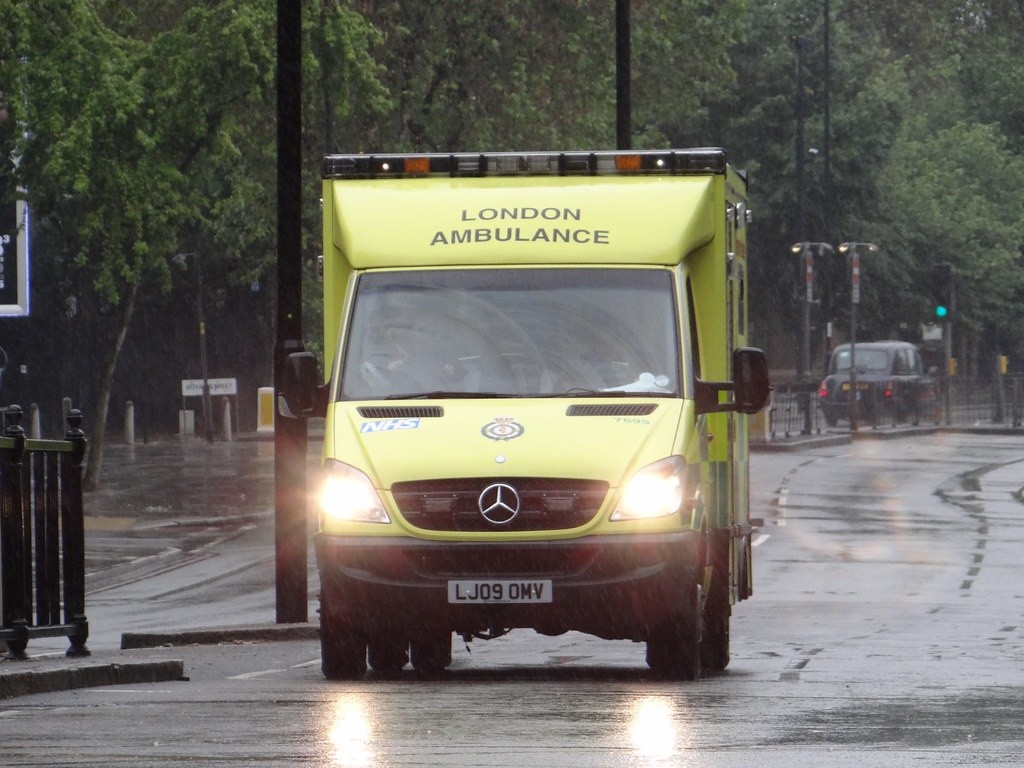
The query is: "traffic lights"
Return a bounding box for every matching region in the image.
[933,264,955,321]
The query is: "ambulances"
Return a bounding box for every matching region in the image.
[273,147,769,678]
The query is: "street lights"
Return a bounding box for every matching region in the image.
[838,242,880,430]
[170,252,213,441]
[791,242,834,411]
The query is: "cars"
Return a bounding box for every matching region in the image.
[820,340,938,424]
